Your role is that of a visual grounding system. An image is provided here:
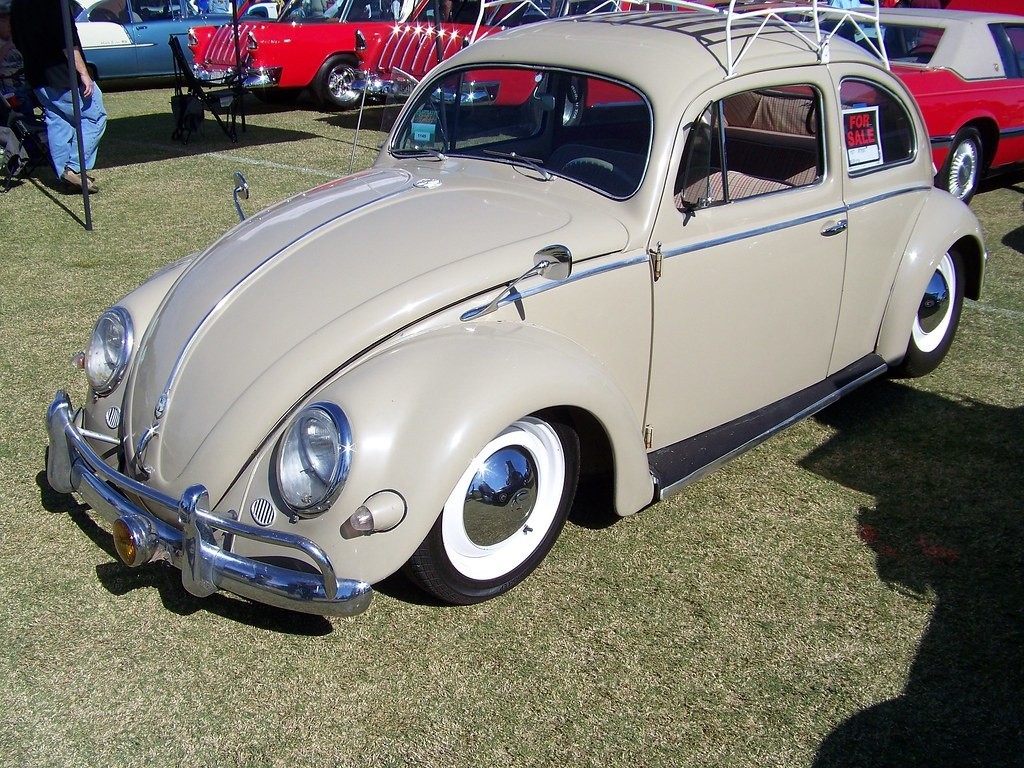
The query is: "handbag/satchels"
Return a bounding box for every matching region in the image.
[172,94,203,131]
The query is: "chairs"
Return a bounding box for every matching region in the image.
[170,34,248,145]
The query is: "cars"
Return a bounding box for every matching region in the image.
[46,12,986,618]
[75,0,1024,206]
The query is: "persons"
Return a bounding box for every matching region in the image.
[307,0,327,16]
[209,0,229,13]
[439,0,452,21]
[9,0,107,192]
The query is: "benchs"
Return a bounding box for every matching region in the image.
[677,89,870,206]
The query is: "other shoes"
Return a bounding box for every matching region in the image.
[61,168,99,194]
